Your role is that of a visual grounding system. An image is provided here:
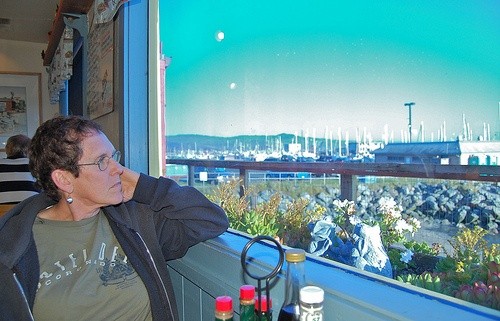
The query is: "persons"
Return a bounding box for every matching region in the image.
[0,135,47,204]
[0,116,230,321]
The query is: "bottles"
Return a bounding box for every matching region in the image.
[278,248,307,321]
[253,295,273,321]
[299,285,325,321]
[214,296,234,321]
[238,285,255,321]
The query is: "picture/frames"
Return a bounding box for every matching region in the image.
[0,71,43,152]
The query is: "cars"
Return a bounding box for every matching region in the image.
[280,153,347,164]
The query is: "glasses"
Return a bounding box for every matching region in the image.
[77,150,122,171]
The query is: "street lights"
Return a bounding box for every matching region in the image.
[403,102,417,144]
